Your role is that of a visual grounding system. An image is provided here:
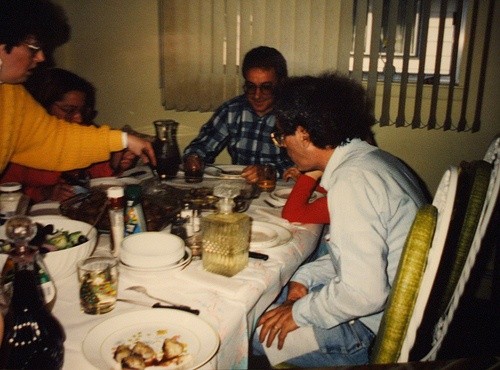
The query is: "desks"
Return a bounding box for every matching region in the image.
[33,165,324,370]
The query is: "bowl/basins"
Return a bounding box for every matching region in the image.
[0,218,97,280]
[271,188,293,201]
[120,233,186,267]
[253,226,279,246]
[216,166,247,178]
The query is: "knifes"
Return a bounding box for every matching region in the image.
[117,298,190,312]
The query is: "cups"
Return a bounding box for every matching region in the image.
[77,257,119,316]
[257,162,276,193]
[183,155,203,183]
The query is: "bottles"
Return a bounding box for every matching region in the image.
[0,182,66,370]
[171,202,201,240]
[202,183,252,276]
[107,186,147,256]
[152,119,180,179]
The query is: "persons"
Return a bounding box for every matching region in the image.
[0,0,157,204]
[251,70,428,370]
[183,46,294,184]
[280,166,332,256]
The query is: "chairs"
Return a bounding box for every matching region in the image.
[374,133,500,366]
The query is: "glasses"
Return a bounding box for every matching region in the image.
[53,101,97,122]
[243,82,275,95]
[271,128,296,148]
[16,36,48,56]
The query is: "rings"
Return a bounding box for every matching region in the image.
[274,326,280,331]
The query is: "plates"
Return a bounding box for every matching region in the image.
[250,221,293,251]
[203,164,249,180]
[82,308,220,370]
[264,185,323,207]
[120,245,192,275]
[86,176,142,186]
